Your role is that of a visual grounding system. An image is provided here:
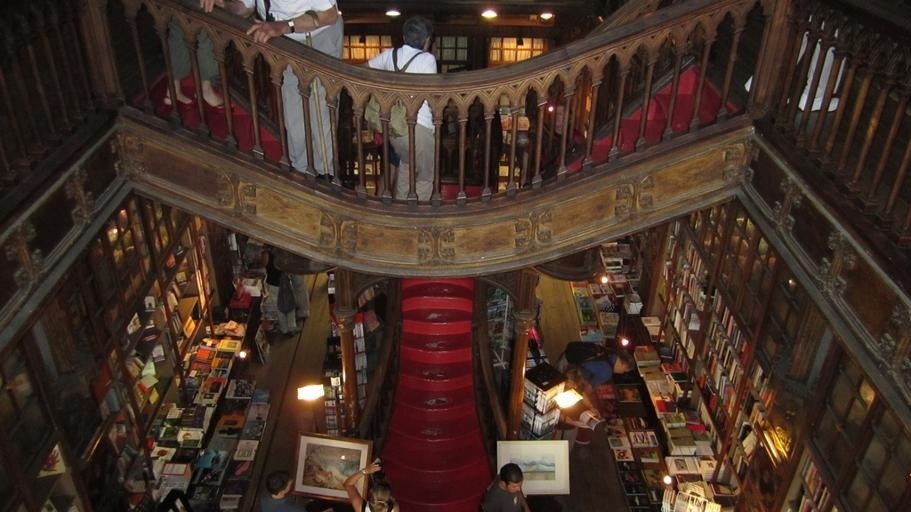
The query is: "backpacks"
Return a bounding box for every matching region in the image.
[365,48,428,137]
[564,341,610,365]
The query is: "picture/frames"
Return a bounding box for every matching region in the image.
[497,439,571,495]
[289,429,374,504]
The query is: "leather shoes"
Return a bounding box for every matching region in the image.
[287,326,302,338]
[164,96,196,109]
[195,93,235,111]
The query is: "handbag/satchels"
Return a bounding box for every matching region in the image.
[278,281,295,312]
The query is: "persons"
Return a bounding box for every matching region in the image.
[163,0,235,113]
[200,0,344,184]
[374,133,399,196]
[361,14,437,201]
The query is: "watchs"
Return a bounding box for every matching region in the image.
[287,19,295,34]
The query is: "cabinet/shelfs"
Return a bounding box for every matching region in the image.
[620,198,909,512]
[1,193,224,510]
